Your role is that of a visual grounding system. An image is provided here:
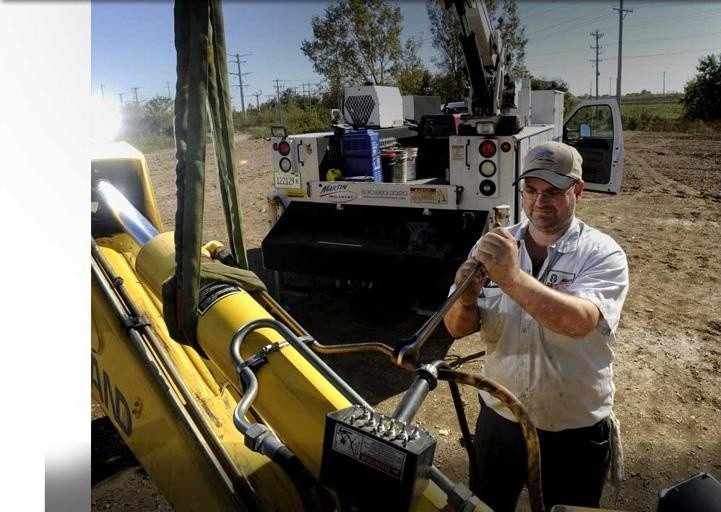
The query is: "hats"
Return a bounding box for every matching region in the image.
[512,140,585,191]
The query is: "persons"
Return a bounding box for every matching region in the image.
[444,141,629,512]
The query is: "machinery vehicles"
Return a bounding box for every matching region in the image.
[224,1,630,314]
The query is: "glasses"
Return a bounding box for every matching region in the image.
[518,183,577,202]
